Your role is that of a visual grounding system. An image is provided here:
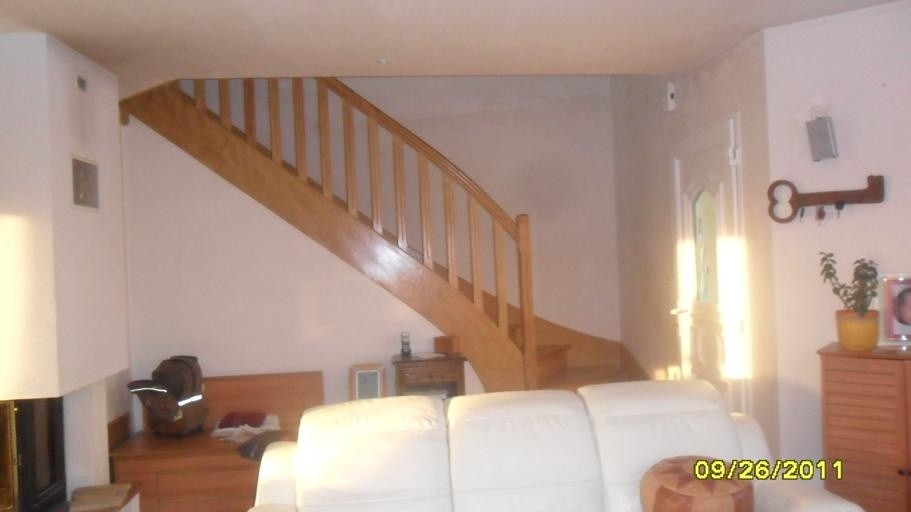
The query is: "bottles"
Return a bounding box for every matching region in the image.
[400,332,411,357]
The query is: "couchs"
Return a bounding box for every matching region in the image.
[246,378,852,512]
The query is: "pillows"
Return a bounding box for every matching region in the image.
[639,455,754,512]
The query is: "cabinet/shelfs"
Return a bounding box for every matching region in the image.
[112,371,323,512]
[815,341,911,511]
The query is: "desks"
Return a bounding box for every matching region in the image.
[392,353,468,396]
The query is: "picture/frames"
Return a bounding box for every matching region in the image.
[880,275,910,352]
[348,363,388,401]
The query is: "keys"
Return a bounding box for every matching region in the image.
[767,175,885,223]
[799,199,844,223]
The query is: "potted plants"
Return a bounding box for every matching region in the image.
[819,249,879,354]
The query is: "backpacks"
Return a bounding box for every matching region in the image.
[126,355,208,438]
